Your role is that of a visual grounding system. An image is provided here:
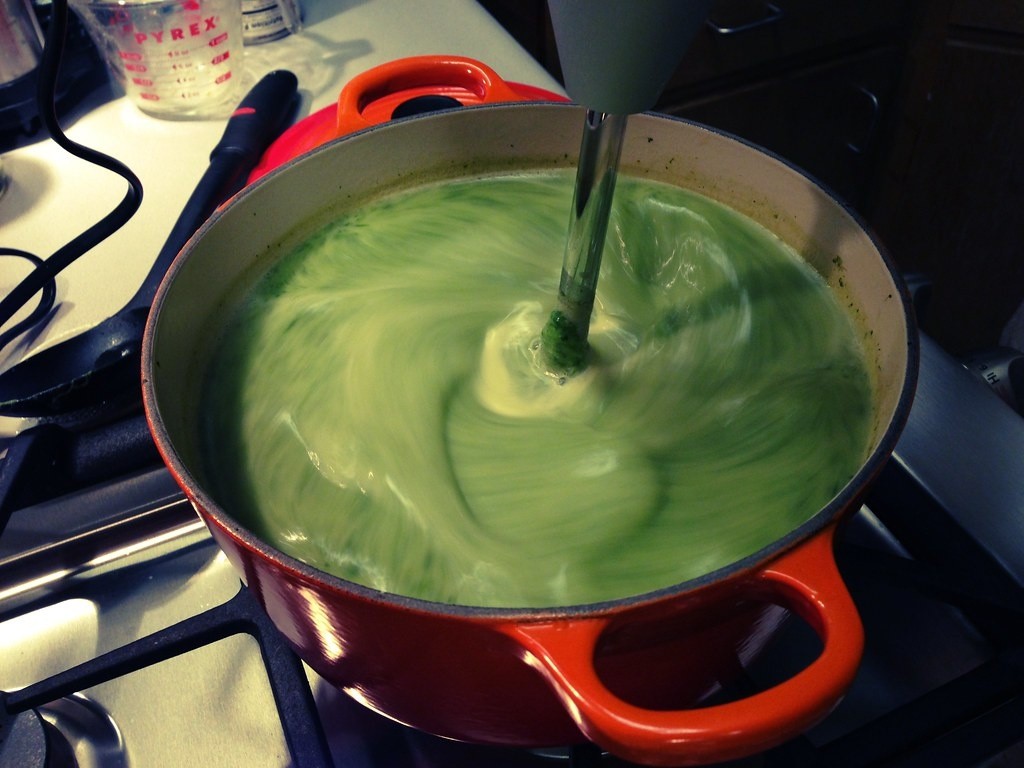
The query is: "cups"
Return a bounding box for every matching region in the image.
[71,0,245,113]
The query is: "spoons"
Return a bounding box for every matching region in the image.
[0,70,298,417]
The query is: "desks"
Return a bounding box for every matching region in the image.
[0,0,571,768]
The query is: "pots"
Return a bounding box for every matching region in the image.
[140,56,920,768]
